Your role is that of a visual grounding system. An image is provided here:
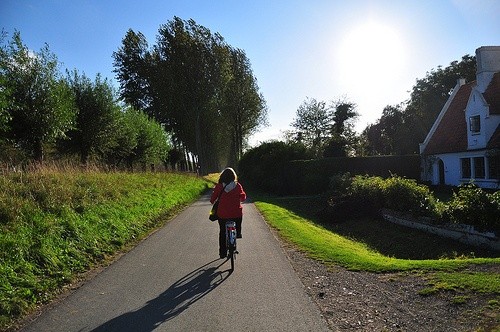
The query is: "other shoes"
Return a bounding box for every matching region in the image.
[236,233,242,238]
[219,250,227,258]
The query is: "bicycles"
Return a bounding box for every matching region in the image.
[217,219,240,272]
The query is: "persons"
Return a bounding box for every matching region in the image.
[210,168,247,259]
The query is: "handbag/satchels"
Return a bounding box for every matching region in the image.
[208,200,218,222]
